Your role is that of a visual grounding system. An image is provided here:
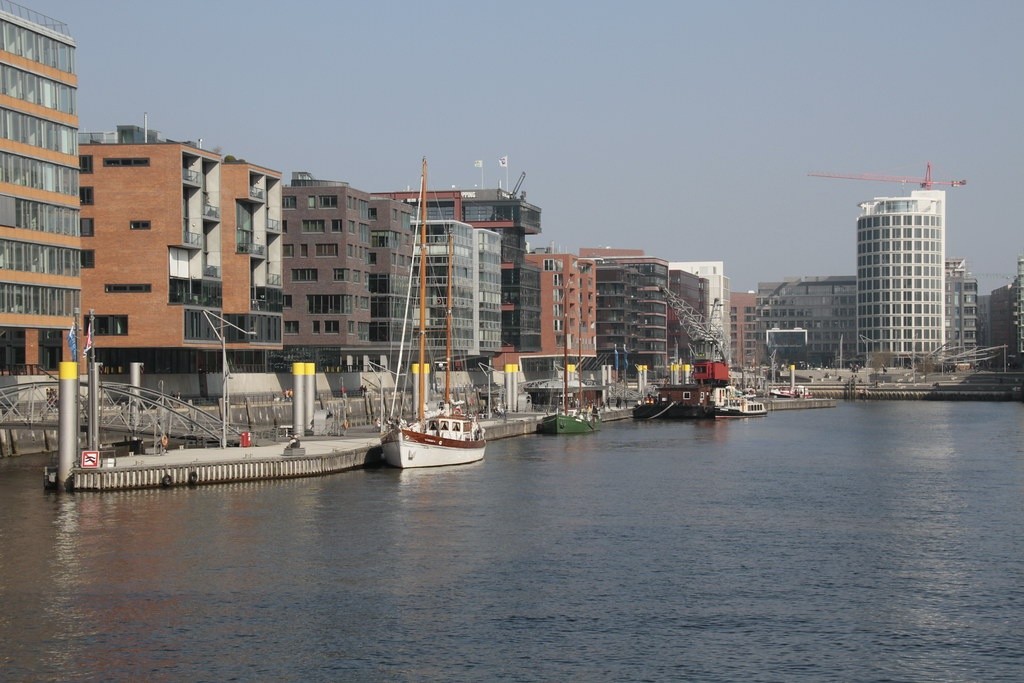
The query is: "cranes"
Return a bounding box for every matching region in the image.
[807,160,968,191]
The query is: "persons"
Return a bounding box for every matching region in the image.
[360,384,363,397]
[283,387,293,402]
[374,412,461,431]
[591,394,674,414]
[362,385,367,397]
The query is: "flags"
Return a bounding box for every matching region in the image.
[82,322,92,359]
[474,159,482,168]
[498,156,507,168]
[65,321,77,362]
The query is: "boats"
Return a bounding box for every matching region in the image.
[632,284,770,419]
[541,313,602,434]
[380,155,486,470]
[769,385,815,399]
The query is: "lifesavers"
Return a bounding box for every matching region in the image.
[452,408,462,416]
[162,435,168,446]
[343,420,349,429]
